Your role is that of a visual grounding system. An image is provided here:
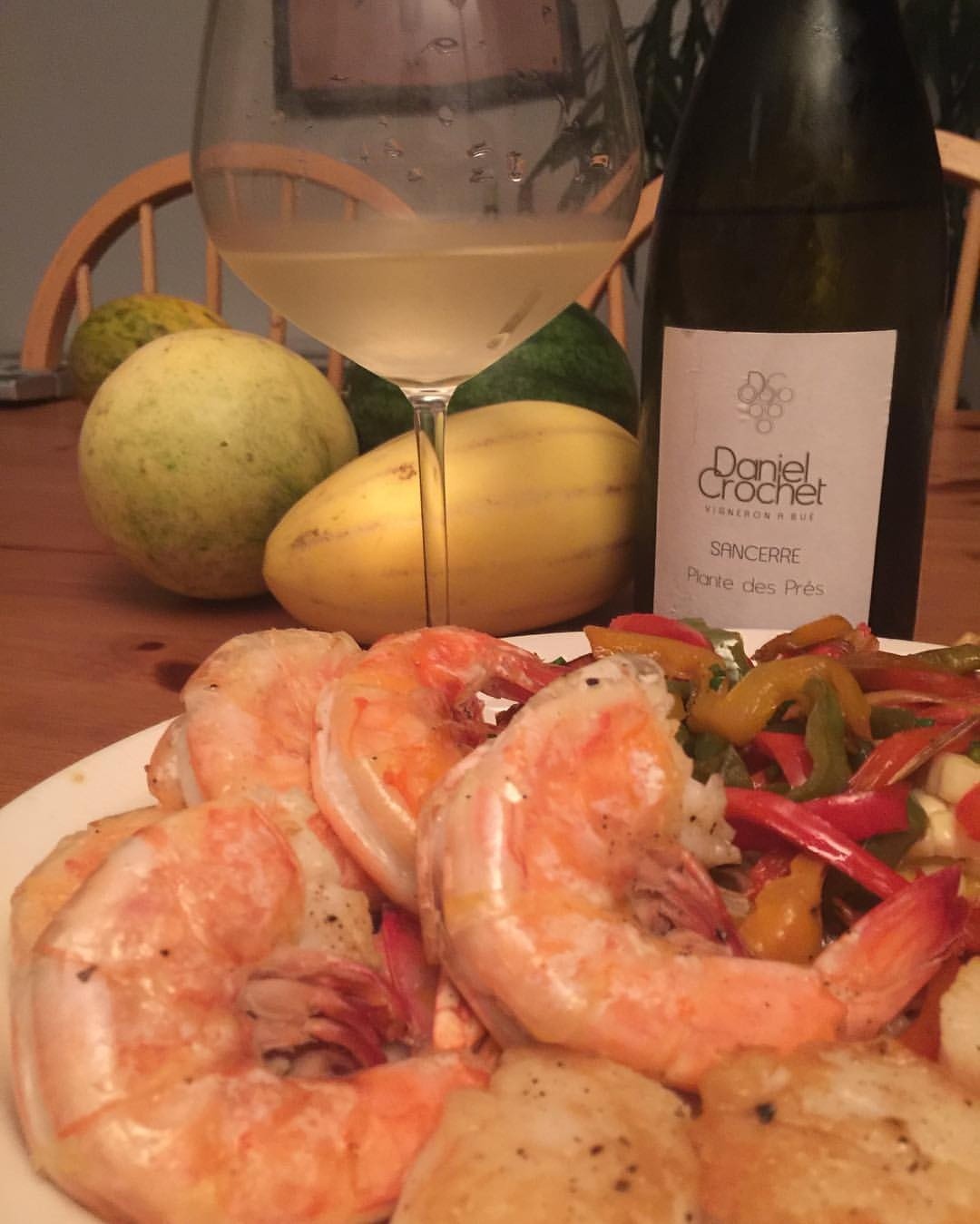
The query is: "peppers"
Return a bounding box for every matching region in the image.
[579,607,980,984]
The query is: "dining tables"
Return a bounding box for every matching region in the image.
[0,373,979,1224]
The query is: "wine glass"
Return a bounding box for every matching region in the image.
[191,0,644,628]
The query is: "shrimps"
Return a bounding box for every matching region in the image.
[5,630,979,1224]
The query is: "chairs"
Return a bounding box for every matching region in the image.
[20,146,420,397]
[551,126,980,414]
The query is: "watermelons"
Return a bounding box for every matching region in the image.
[332,299,640,461]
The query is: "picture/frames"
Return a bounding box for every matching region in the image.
[275,0,588,119]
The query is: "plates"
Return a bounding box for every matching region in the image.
[2,626,980,1224]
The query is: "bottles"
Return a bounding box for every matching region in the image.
[642,0,952,644]
